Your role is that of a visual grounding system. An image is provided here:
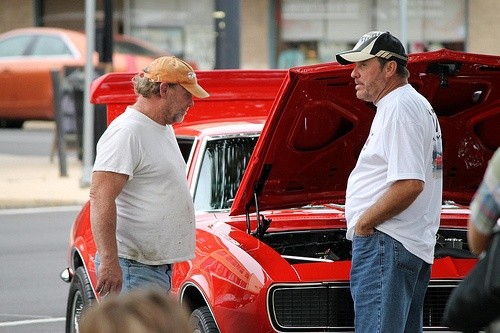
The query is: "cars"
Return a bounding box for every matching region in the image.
[0,24,198,127]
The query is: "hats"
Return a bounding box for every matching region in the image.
[336,30,408,68]
[138,55,211,98]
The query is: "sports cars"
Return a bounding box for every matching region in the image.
[58,44,496,332]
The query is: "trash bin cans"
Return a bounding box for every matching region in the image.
[72,73,107,164]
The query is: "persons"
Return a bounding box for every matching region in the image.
[76,55,209,333]
[337,30,443,333]
[468,145,500,333]
